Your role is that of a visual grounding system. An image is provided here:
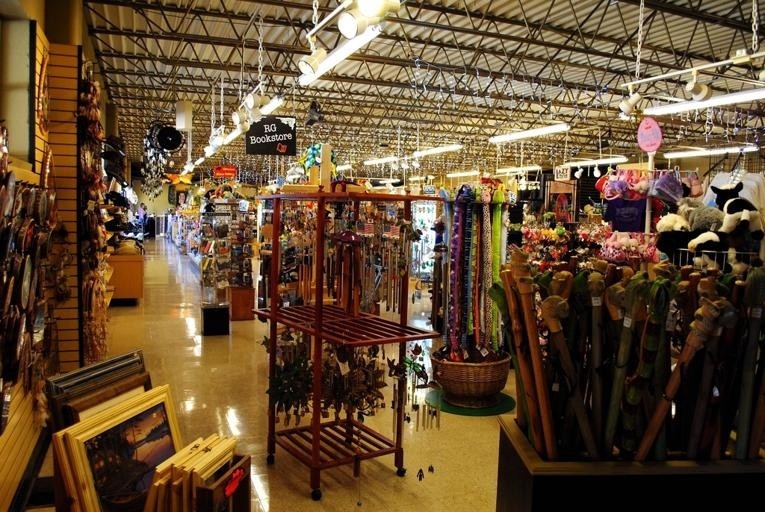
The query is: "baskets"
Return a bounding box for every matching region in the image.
[430,347,512,409]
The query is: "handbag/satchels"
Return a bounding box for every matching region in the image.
[646,165,703,202]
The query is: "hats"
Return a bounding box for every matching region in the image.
[102,134,125,194]
[116,240,138,255]
[105,191,127,232]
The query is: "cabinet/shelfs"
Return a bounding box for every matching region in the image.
[251,185,445,501]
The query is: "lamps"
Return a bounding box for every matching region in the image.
[172,0,401,174]
[619,70,712,115]
[488,122,572,143]
[401,158,422,170]
[663,144,759,160]
[496,164,541,178]
[408,169,484,180]
[611,86,765,117]
[559,155,629,180]
[412,138,463,156]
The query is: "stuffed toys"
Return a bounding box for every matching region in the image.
[595,166,765,276]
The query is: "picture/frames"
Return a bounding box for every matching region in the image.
[45,349,238,512]
